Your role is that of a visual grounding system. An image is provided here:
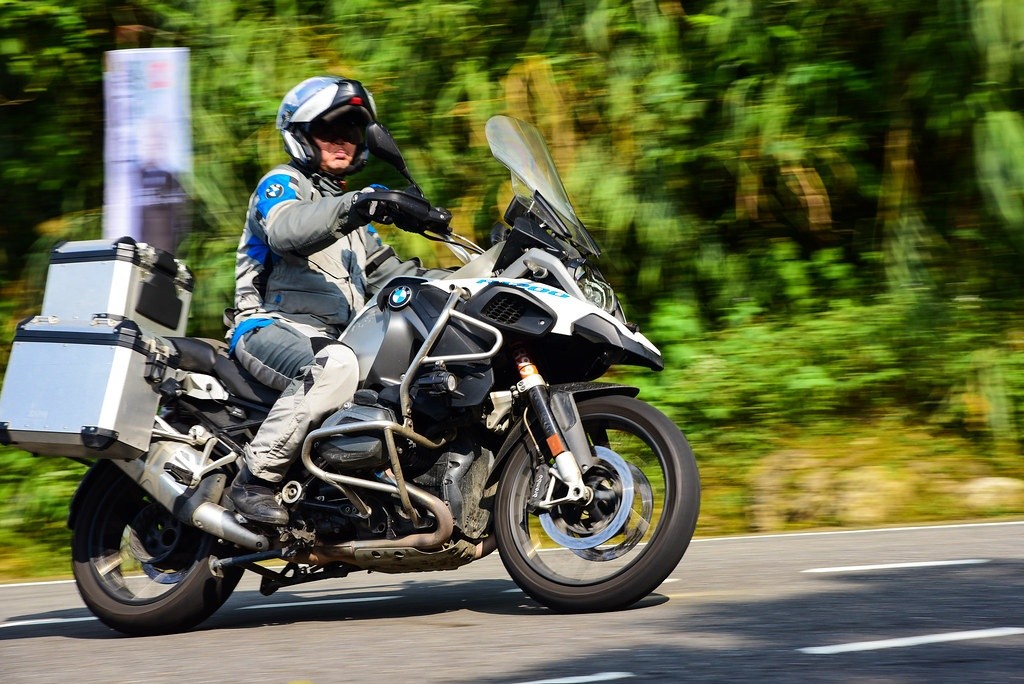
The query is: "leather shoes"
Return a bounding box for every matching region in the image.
[227,463,289,524]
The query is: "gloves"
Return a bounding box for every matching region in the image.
[352,181,395,225]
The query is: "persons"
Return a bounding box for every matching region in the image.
[223,75,462,524]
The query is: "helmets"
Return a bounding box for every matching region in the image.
[274,74,376,175]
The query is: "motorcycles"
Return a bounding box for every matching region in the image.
[65,113,703,638]
